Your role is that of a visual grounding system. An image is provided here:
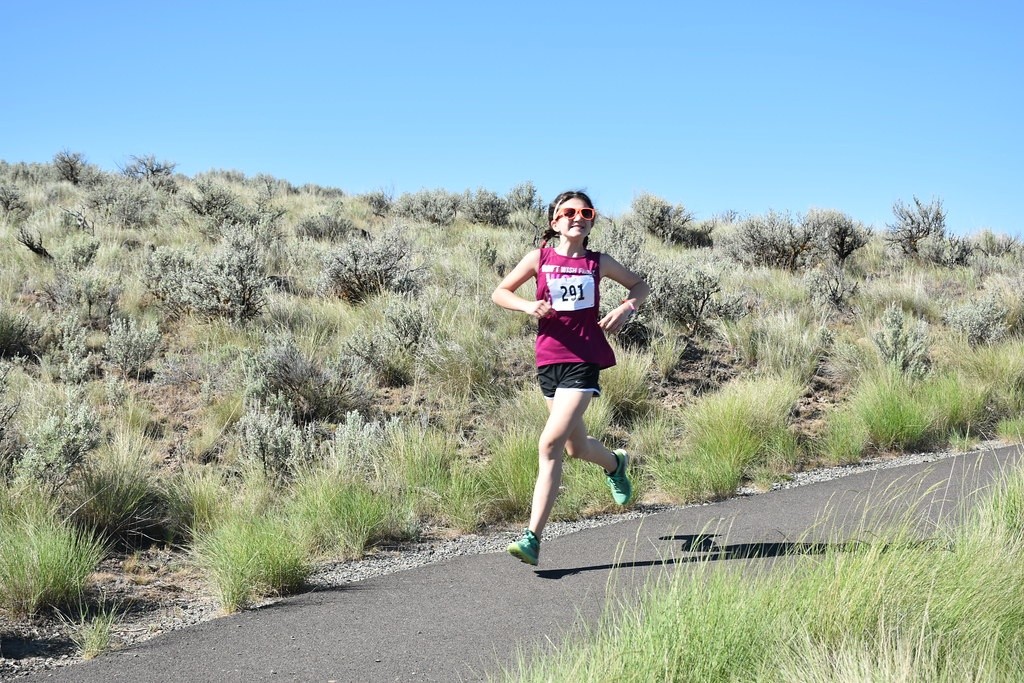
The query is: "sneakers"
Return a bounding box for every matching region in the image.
[603,449,631,506]
[508,529,540,566]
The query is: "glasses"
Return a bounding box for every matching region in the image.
[554,208,596,221]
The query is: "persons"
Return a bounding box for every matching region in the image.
[492,191,650,566]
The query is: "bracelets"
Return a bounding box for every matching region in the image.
[622,299,636,317]
